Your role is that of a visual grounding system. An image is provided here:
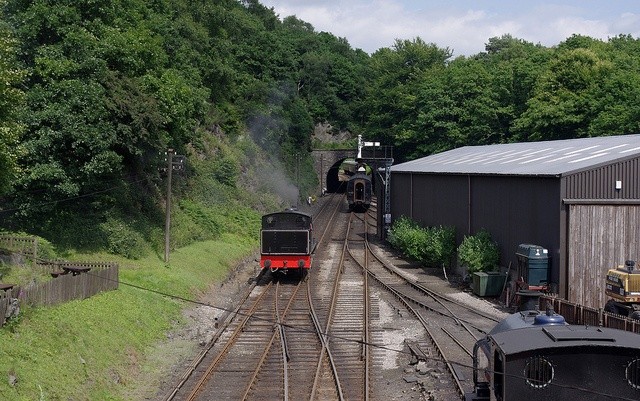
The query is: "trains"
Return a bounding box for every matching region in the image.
[473,304,640,401]
[346,176,373,212]
[259,205,311,280]
